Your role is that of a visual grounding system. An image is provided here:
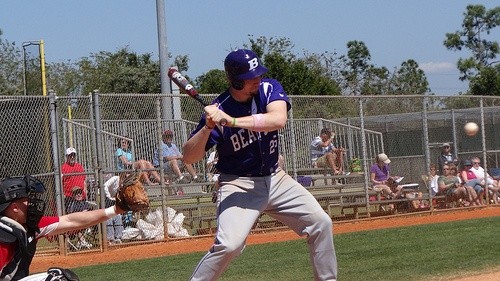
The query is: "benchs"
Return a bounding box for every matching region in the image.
[97,166,446,235]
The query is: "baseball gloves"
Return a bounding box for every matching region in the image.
[113,171,149,216]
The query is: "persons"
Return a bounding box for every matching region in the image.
[430,163,440,197]
[183,49,338,281]
[438,157,500,205]
[369,153,404,209]
[153,130,204,184]
[207,150,219,174]
[0,172,149,281]
[438,142,458,174]
[115,136,170,186]
[311,128,346,175]
[103,171,131,245]
[61,148,87,213]
[65,186,92,248]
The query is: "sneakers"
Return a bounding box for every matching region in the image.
[193,177,203,183]
[178,176,189,183]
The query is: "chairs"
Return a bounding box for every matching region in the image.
[420,171,500,207]
[67,228,99,252]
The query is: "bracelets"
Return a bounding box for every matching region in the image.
[105,206,119,219]
[331,135,334,138]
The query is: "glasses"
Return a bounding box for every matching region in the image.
[444,146,449,149]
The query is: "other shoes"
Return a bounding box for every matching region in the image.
[77,241,92,248]
[391,188,403,200]
[334,171,350,175]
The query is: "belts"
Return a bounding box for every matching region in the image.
[221,163,278,177]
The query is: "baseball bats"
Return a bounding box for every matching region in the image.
[167,67,227,127]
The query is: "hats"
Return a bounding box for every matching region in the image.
[224,49,267,80]
[464,161,473,166]
[0,177,44,213]
[377,153,391,165]
[442,143,450,147]
[72,186,83,195]
[162,131,173,138]
[65,148,76,158]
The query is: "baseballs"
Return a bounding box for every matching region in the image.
[462,122,480,137]
